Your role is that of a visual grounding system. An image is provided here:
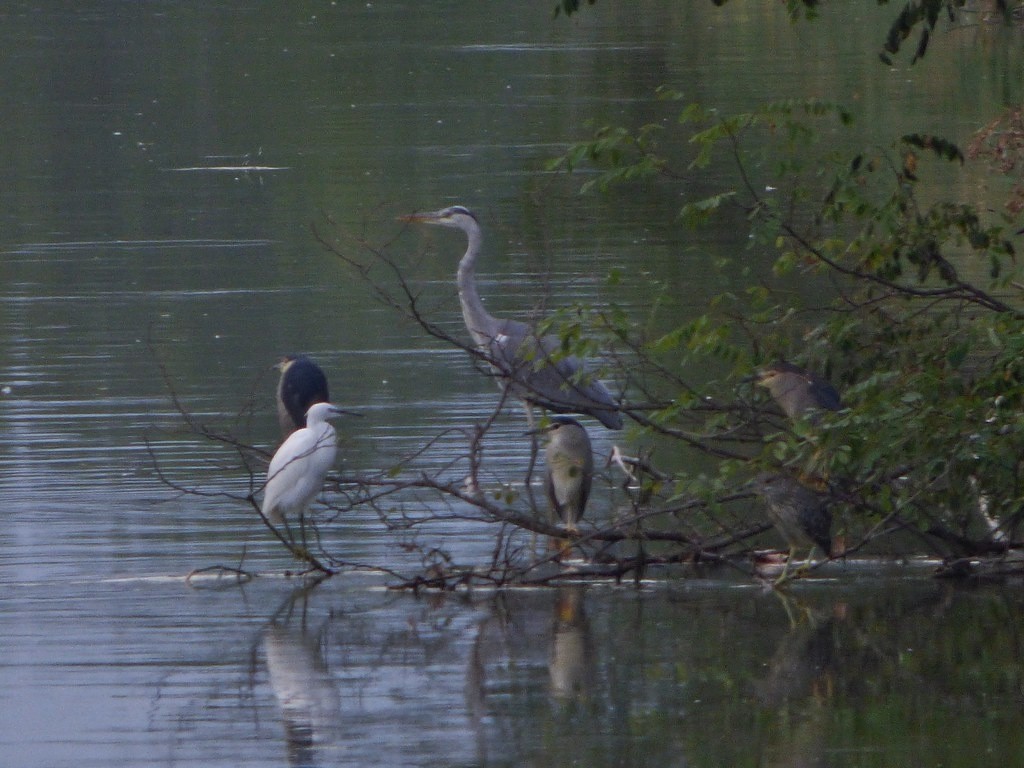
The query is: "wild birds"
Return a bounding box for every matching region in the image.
[261,206,849,592]
